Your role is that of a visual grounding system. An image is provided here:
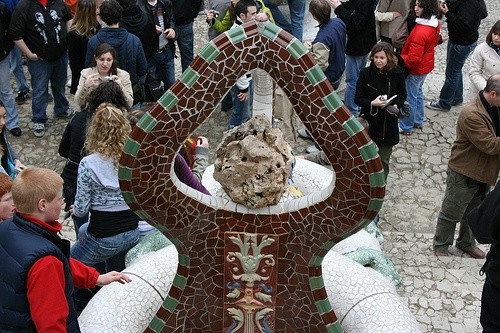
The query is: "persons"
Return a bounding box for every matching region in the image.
[74,43,134,108]
[296,0,488,176]
[204,0,305,129]
[432,19,500,333]
[66,103,141,274]
[0,0,76,179]
[0,168,132,333]
[58,79,130,242]
[63,0,205,104]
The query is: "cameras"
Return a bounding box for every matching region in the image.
[103,76,115,83]
[212,10,220,19]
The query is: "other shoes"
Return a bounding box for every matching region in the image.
[397,123,423,135]
[14,90,30,104]
[10,127,22,136]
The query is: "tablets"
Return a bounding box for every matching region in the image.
[381,94,398,109]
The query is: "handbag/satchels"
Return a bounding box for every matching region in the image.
[139,80,165,102]
[467,178,500,245]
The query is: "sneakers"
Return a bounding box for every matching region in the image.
[32,123,45,137]
[426,101,451,111]
[450,99,463,107]
[54,107,78,119]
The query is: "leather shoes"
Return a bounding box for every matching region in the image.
[434,246,449,257]
[455,240,485,259]
